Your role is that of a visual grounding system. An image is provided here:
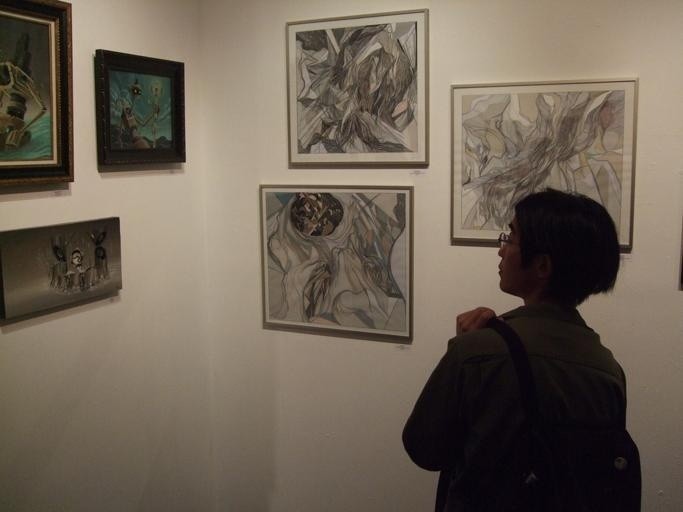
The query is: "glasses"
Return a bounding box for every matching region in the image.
[496,232,519,249]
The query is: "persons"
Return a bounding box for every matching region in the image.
[401,183,643,512]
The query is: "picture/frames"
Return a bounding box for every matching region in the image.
[258,185,414,345]
[451,76,639,255]
[0,0,75,188]
[96,49,186,164]
[285,8,431,164]
